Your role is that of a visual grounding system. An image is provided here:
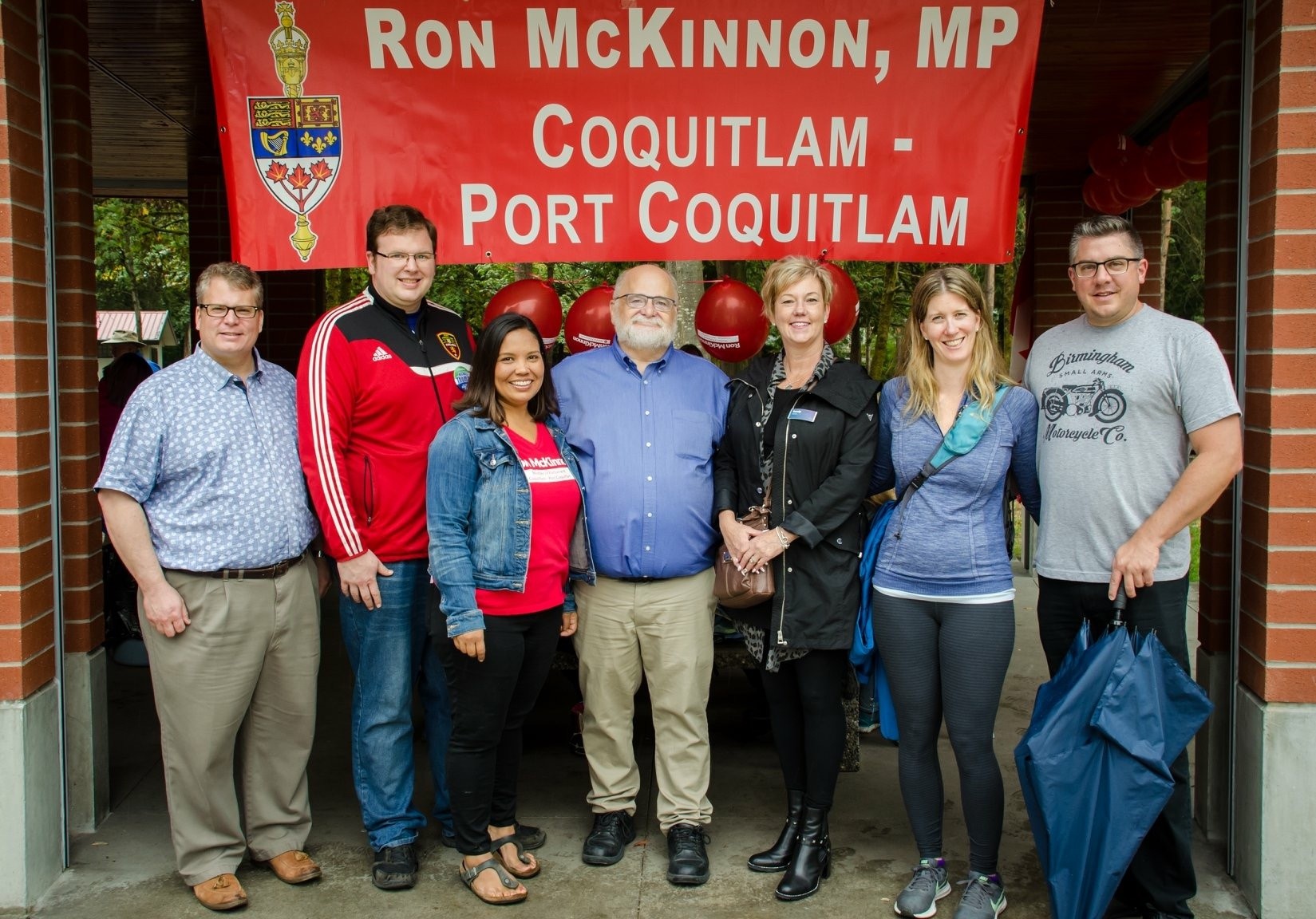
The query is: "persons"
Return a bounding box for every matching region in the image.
[425,313,595,906]
[859,266,1042,919]
[93,261,334,911]
[295,205,476,889]
[97,331,162,637]
[535,264,727,888]
[1022,215,1244,919]
[710,255,884,900]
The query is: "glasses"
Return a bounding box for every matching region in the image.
[616,293,675,312]
[372,249,435,263]
[200,304,262,318]
[1071,258,1140,279]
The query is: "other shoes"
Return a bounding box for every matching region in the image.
[442,821,546,849]
[858,711,880,733]
[371,843,418,889]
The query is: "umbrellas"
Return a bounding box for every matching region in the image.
[1014,576,1214,919]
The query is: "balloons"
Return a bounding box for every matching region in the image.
[1082,99,1210,216]
[812,261,860,344]
[564,284,616,356]
[694,276,770,364]
[483,279,563,351]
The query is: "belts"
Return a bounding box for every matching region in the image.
[173,549,306,579]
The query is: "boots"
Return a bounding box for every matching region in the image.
[747,791,805,873]
[775,804,831,900]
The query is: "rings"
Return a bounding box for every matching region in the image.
[751,556,757,563]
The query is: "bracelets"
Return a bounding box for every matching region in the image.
[312,549,324,558]
[779,528,790,549]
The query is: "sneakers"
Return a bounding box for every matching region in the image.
[954,870,1007,919]
[894,857,951,919]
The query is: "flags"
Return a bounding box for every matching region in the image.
[1007,213,1035,384]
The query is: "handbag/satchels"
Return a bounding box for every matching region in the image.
[713,497,774,609]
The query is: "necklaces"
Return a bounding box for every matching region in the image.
[783,357,814,390]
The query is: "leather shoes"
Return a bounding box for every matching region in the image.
[583,808,635,865]
[193,873,248,910]
[251,850,322,884]
[666,823,710,884]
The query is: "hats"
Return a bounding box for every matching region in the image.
[100,330,148,348]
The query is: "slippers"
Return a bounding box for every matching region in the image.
[459,856,528,903]
[489,834,540,879]
